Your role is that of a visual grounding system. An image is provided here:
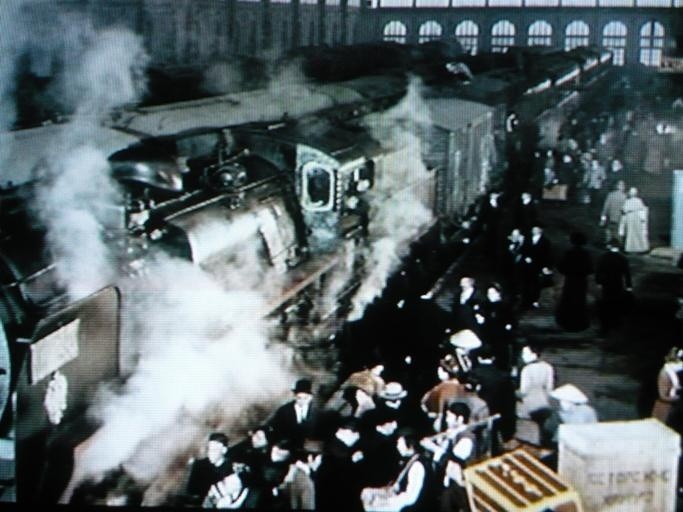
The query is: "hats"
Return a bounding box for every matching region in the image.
[378,382,408,400]
[292,380,312,394]
[549,383,589,404]
[296,439,325,455]
[450,329,482,350]
